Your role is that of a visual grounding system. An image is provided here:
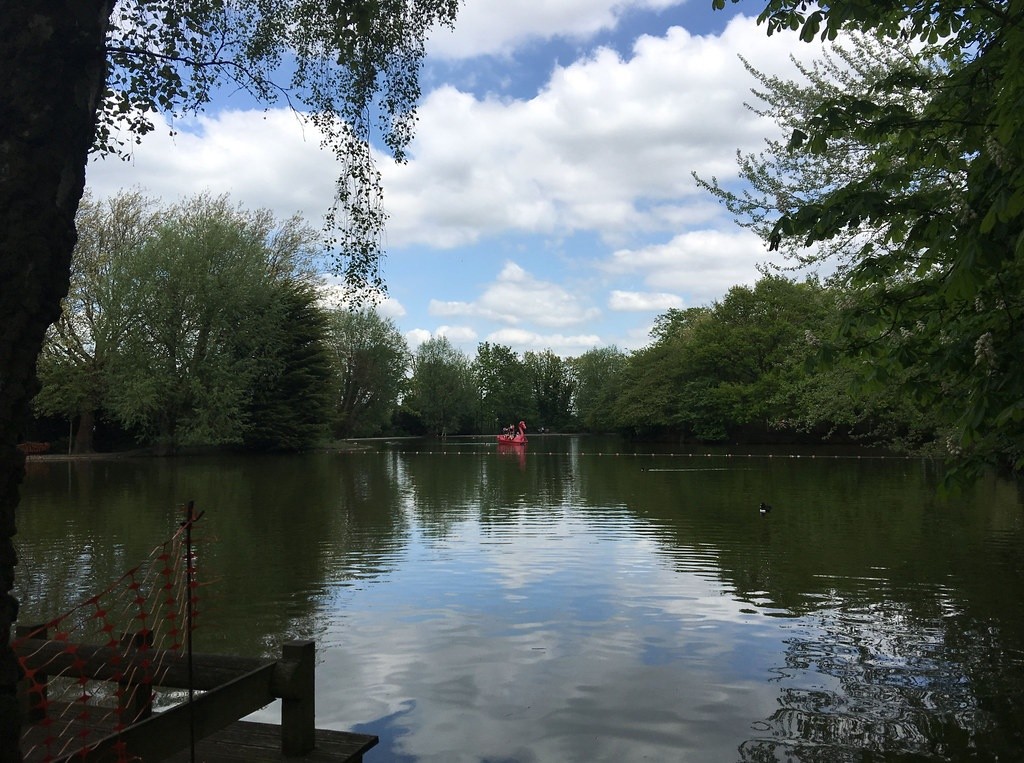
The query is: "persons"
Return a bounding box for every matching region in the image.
[441,425,447,436]
[503,424,519,439]
[542,426,545,434]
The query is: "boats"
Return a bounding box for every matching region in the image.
[497,420,529,444]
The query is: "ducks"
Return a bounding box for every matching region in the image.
[759,503,772,514]
[496,421,529,443]
